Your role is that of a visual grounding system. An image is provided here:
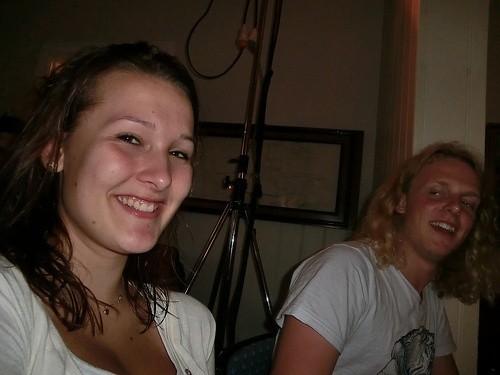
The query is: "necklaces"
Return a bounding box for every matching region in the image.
[88,294,123,314]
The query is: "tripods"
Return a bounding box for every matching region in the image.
[184,0,276,358]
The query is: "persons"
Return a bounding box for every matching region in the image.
[0,39,216,375]
[271,140,500,375]
